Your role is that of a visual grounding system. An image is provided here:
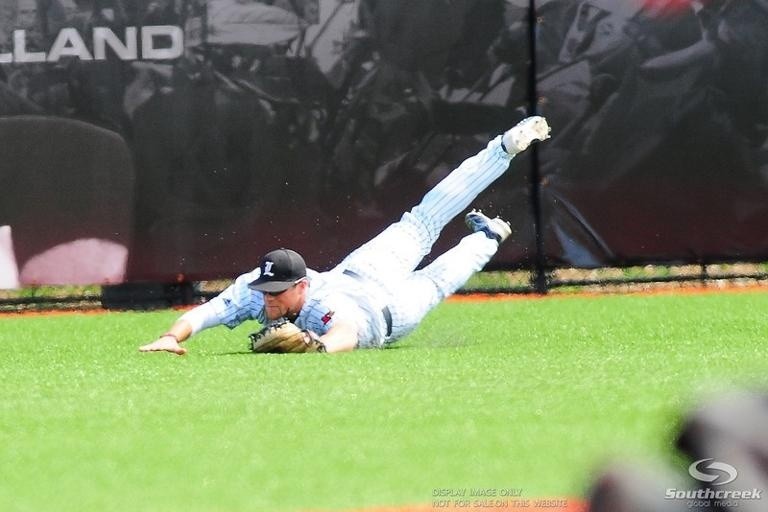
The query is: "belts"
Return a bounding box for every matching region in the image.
[341,268,393,339]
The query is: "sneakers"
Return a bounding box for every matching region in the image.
[464,207,514,246]
[499,116,554,156]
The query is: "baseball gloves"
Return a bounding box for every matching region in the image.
[248,318,325,354]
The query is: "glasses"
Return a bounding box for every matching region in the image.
[261,280,304,297]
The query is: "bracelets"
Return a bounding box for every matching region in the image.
[159,333,179,342]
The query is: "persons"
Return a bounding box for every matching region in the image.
[137,113,555,355]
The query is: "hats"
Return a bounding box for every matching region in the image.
[247,247,307,293]
